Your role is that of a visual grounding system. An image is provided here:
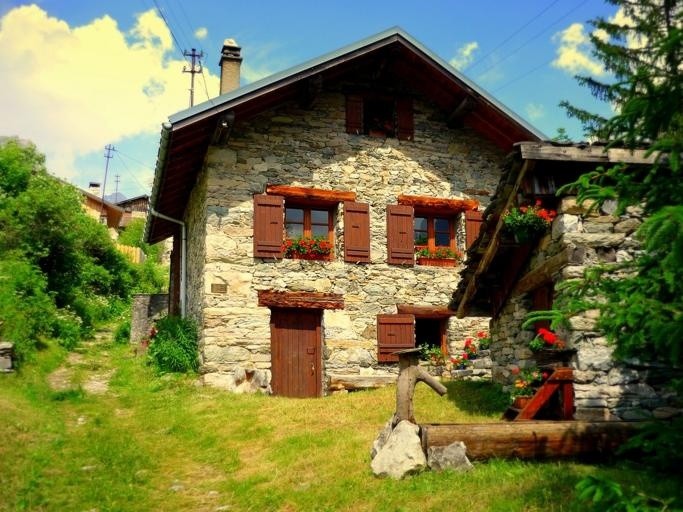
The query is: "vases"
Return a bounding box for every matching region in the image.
[292,251,335,260]
[457,364,465,369]
[481,345,488,350]
[470,354,477,359]
[418,256,456,267]
[515,397,533,409]
[514,227,534,244]
[533,350,562,367]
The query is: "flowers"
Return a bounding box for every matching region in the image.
[416,245,464,260]
[282,239,334,255]
[477,332,489,344]
[451,354,470,366]
[465,339,476,357]
[502,368,549,405]
[502,199,557,230]
[529,328,566,351]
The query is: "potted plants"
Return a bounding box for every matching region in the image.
[429,345,440,361]
